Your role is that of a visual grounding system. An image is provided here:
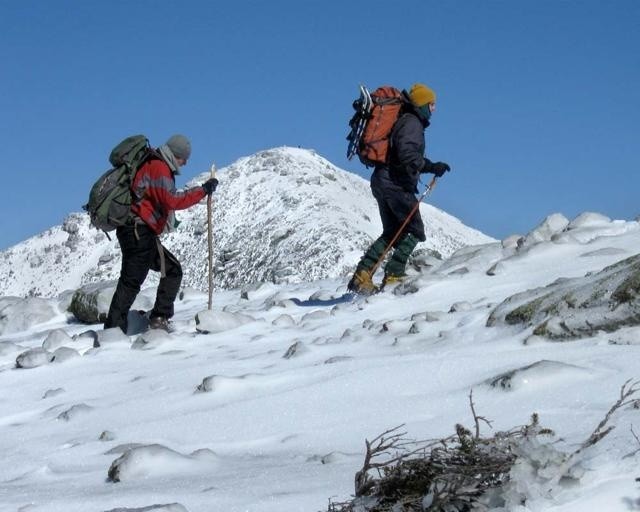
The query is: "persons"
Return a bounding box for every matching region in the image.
[348,83,450,294]
[100,134,219,334]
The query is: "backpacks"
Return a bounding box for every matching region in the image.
[346,84,405,170]
[82,134,164,240]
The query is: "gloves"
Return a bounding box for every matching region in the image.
[421,158,450,177]
[202,178,218,194]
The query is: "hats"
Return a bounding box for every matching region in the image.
[409,84,435,108]
[167,135,192,159]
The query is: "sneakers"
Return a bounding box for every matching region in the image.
[149,316,174,334]
[348,270,378,296]
